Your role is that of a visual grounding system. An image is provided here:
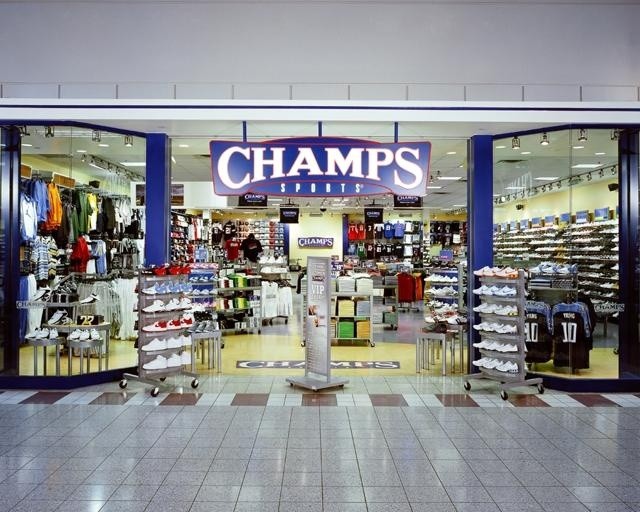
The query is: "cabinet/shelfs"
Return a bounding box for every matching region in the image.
[17,163,619,400]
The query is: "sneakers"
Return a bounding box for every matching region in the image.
[30,285,51,301]
[78,293,99,305]
[66,328,101,341]
[141,262,218,371]
[493,219,619,304]
[424,260,469,333]
[24,327,60,340]
[472,266,524,373]
[47,309,72,326]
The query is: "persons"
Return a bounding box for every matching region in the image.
[223,233,262,263]
